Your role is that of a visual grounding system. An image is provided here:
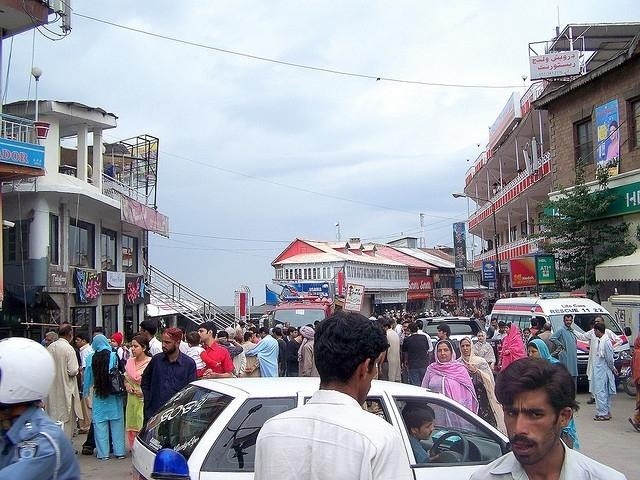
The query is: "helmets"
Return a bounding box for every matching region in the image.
[0,336,56,404]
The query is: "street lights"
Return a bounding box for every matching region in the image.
[449,191,501,298]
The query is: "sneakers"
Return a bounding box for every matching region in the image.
[82,445,95,456]
[586,397,596,405]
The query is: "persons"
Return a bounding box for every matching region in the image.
[629,336,640,431]
[0,337,81,478]
[39,310,619,463]
[255,311,414,479]
[470,355,626,478]
[607,121,619,159]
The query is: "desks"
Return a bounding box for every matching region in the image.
[59,164,76,177]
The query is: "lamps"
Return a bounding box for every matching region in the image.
[521,75,529,90]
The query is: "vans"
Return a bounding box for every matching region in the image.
[487,290,632,387]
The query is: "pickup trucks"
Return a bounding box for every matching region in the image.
[410,312,502,370]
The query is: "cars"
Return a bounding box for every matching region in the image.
[128,374,515,480]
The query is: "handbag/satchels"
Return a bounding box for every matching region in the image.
[109,352,127,395]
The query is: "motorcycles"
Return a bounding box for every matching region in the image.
[613,349,637,397]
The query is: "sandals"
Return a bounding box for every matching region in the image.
[628,416,640,432]
[592,414,613,421]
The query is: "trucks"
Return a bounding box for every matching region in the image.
[271,289,337,331]
